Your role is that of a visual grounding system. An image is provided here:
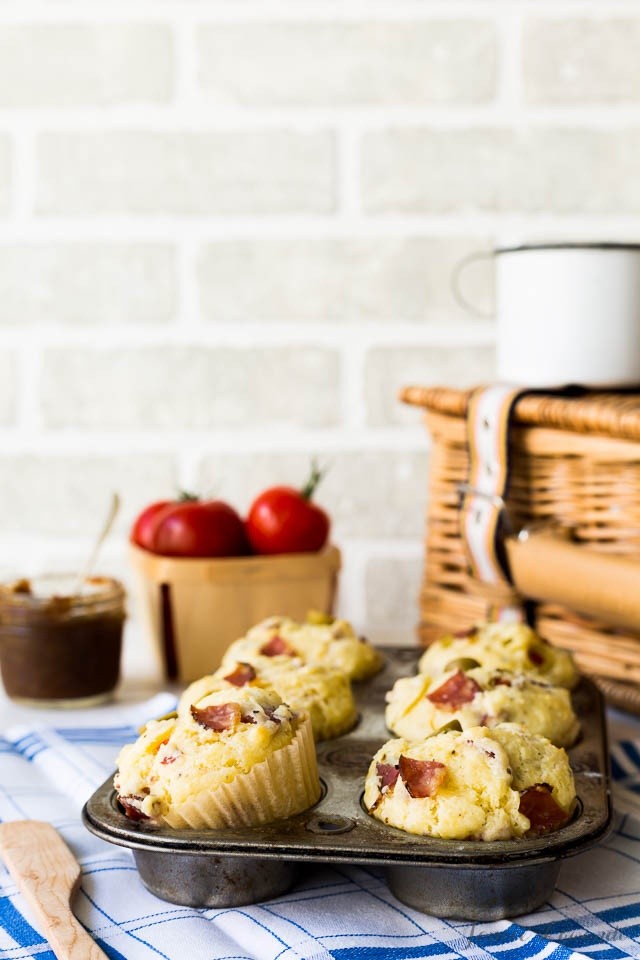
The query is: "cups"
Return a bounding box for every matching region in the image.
[449,242,639,390]
[129,534,340,688]
[1,575,128,705]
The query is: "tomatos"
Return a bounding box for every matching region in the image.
[130,461,331,559]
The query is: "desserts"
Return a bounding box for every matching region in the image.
[108,613,585,841]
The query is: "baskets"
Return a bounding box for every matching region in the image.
[393,379,639,717]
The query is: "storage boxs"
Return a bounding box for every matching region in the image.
[398,385,639,717]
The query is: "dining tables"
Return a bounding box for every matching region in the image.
[1,688,640,959]
